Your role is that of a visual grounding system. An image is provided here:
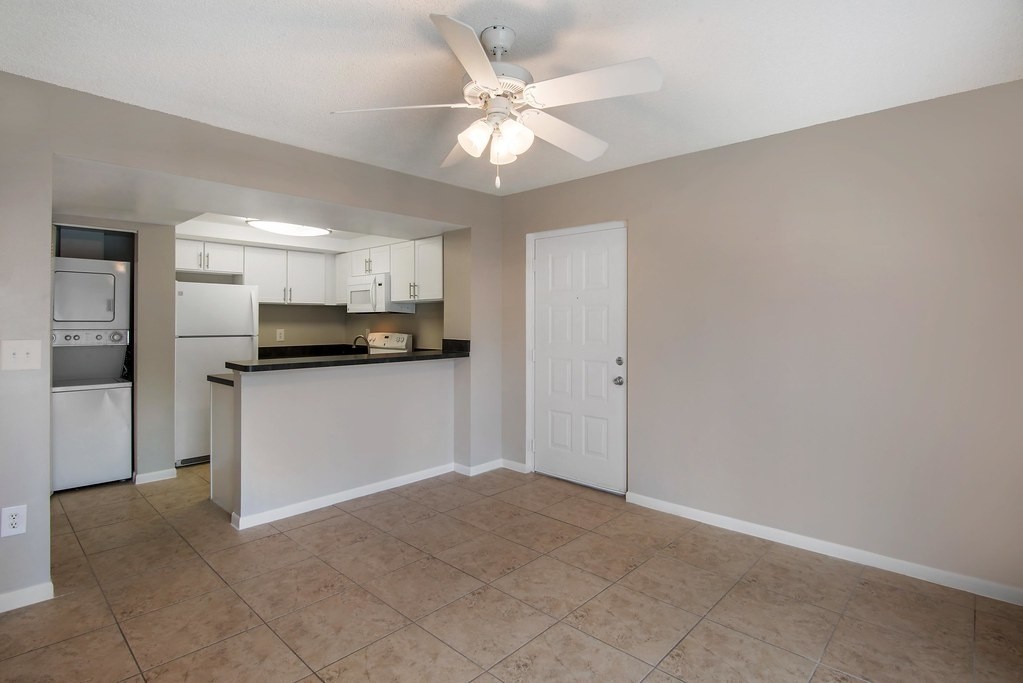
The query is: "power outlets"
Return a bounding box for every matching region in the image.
[1,504,26,537]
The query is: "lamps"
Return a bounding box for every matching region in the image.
[458,96,535,189]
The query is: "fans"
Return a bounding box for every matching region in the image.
[332,14,662,170]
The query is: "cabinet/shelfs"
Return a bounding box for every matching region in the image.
[337,245,391,305]
[176,239,326,303]
[390,237,443,304]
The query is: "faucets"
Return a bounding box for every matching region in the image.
[352,335,372,355]
